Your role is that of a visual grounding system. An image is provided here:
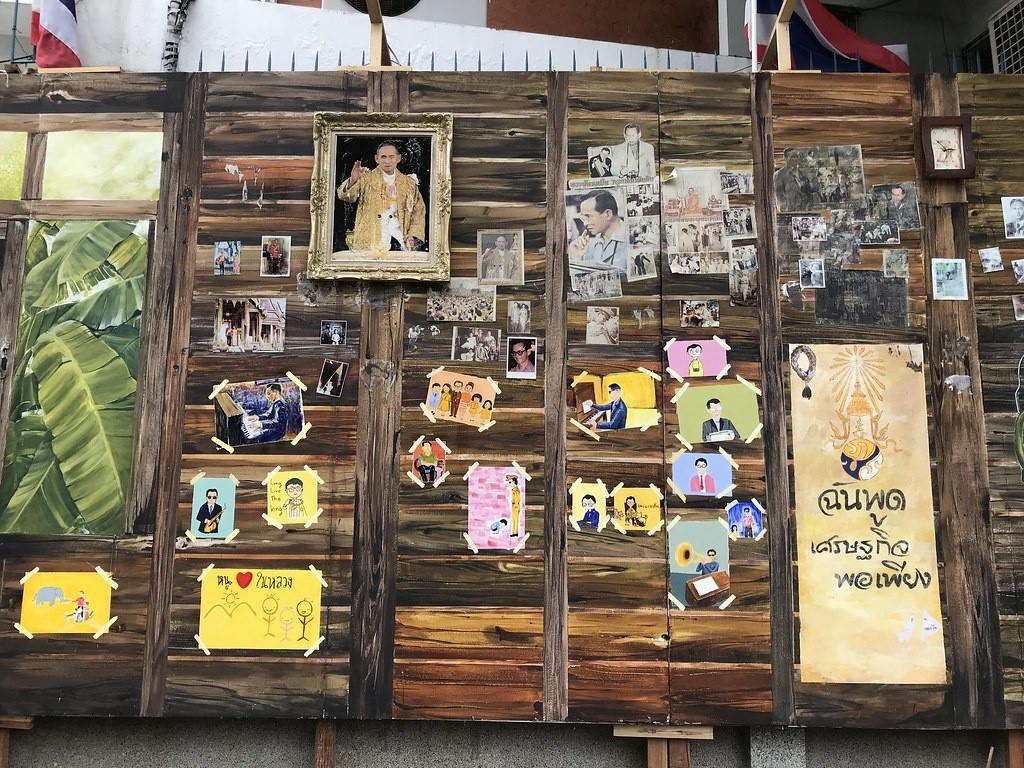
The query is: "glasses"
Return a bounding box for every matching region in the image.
[580,212,601,222]
[512,349,526,356]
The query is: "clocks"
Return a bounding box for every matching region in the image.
[919,114,978,180]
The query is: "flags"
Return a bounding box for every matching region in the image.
[31,0,81,68]
[745,0,909,73]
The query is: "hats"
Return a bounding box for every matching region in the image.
[604,321,618,344]
[595,308,615,321]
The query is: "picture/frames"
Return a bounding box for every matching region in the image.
[306,112,453,283]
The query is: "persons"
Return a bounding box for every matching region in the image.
[671,255,730,272]
[773,148,850,211]
[737,173,746,193]
[679,223,709,252]
[684,187,702,213]
[627,196,654,216]
[233,252,239,275]
[509,339,535,372]
[482,235,518,279]
[454,329,497,360]
[738,272,752,304]
[684,303,715,327]
[881,186,915,228]
[270,238,280,274]
[337,141,426,252]
[731,247,756,270]
[217,250,227,275]
[1006,199,1024,237]
[635,252,651,275]
[629,219,660,245]
[569,190,627,272]
[866,221,892,243]
[510,302,528,332]
[793,218,825,240]
[590,147,613,178]
[331,329,340,344]
[427,294,494,321]
[724,208,752,235]
[588,308,618,344]
